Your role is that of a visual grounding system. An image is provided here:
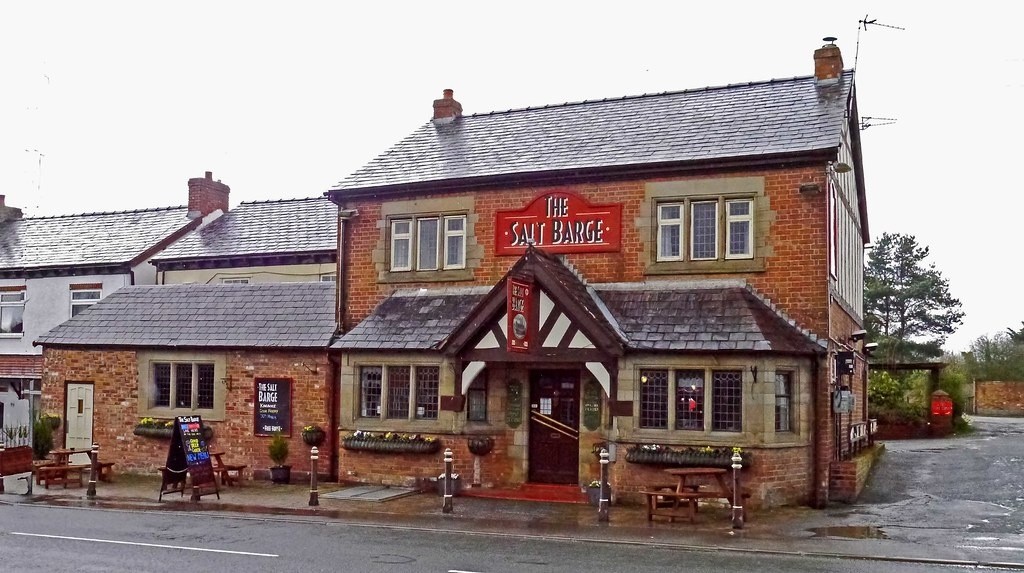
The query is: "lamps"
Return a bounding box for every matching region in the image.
[849,329,867,343]
[834,161,852,174]
[799,183,822,195]
[865,343,879,352]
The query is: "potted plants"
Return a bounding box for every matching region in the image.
[266,429,293,485]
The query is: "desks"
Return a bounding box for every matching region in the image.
[49,448,92,488]
[209,451,235,487]
[665,467,735,523]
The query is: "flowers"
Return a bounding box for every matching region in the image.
[344,429,439,444]
[590,479,611,489]
[626,443,753,460]
[302,424,322,433]
[133,418,174,431]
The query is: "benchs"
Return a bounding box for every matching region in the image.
[33,458,116,488]
[158,464,248,486]
[640,484,751,524]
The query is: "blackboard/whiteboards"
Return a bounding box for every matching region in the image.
[254,377,292,437]
[163,416,215,486]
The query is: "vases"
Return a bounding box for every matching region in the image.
[587,487,612,508]
[343,439,433,453]
[624,450,752,468]
[302,430,325,447]
[133,428,213,439]
